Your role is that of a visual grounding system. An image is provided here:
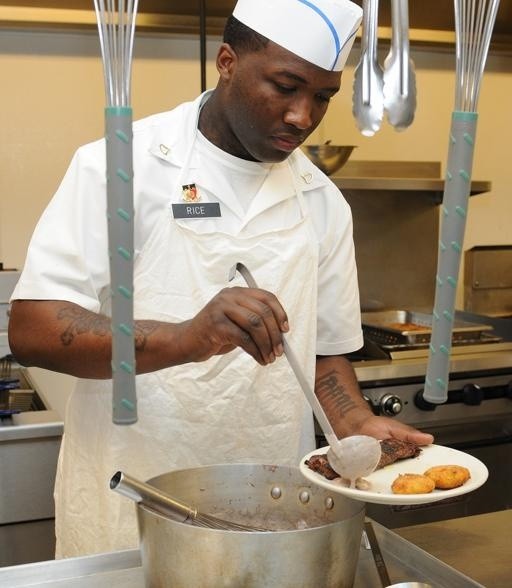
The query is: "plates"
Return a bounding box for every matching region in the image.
[297,437,491,506]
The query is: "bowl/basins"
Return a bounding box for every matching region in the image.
[134,463,366,586]
[301,142,359,176]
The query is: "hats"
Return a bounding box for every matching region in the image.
[234,0,364,72]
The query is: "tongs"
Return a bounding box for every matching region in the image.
[353,1,419,140]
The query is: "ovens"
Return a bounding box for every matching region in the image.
[365,416,512,531]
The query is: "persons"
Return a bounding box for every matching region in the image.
[7,0,437,563]
